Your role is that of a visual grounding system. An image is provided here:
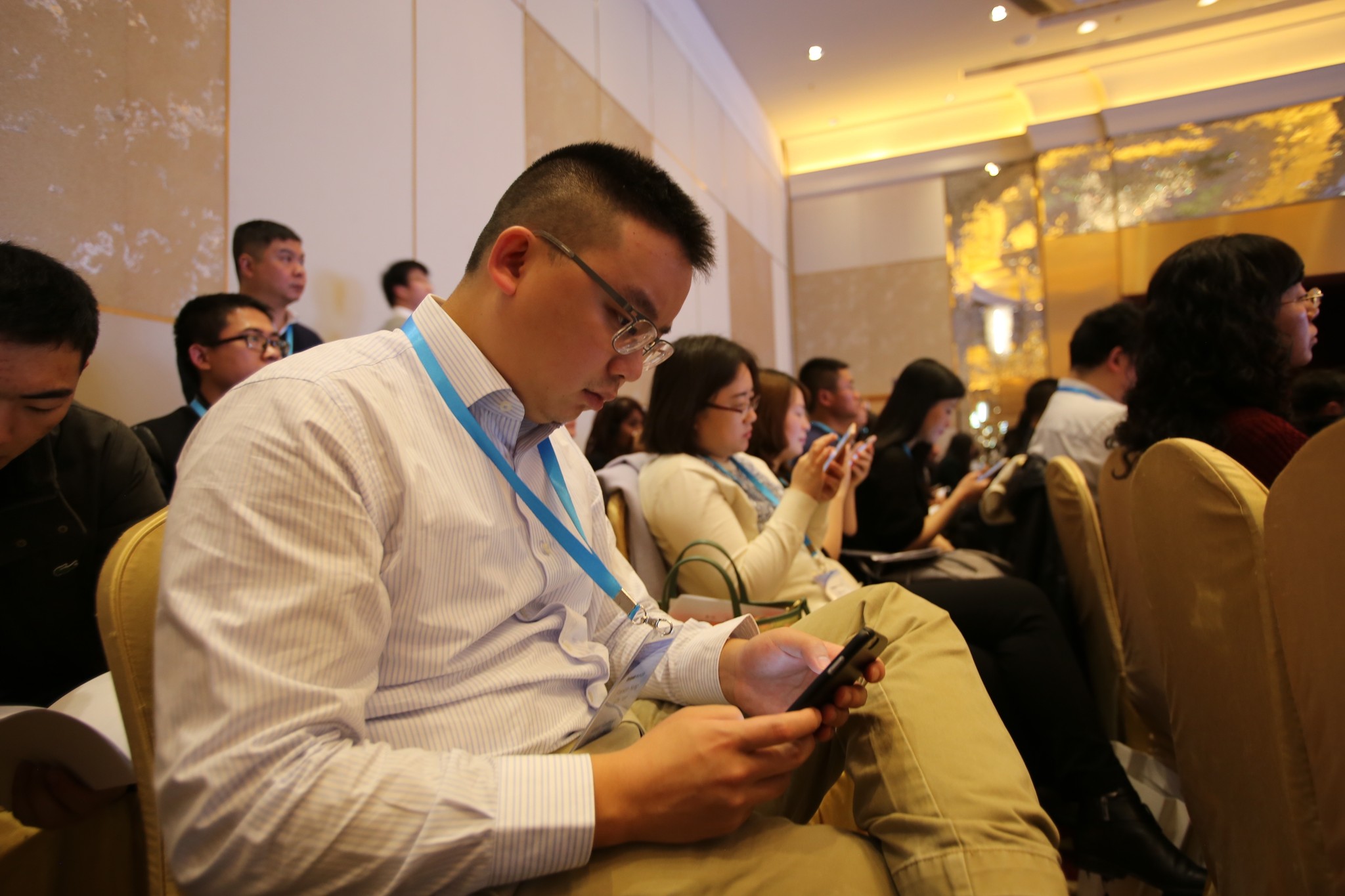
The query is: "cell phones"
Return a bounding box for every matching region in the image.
[847,434,875,466]
[787,626,888,711]
[823,421,856,470]
[977,457,1010,480]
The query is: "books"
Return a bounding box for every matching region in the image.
[0,667,139,790]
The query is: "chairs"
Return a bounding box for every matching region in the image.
[95,504,165,895]
[1043,420,1345,896]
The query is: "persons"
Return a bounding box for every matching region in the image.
[0,240,170,896]
[1102,233,1323,490]
[1026,301,1140,463]
[233,220,325,356]
[584,334,1211,896]
[128,294,290,506]
[152,142,1068,896]
[380,261,434,330]
[1280,364,1345,438]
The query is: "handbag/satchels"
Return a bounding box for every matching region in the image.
[844,548,1015,615]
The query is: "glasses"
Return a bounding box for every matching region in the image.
[210,330,289,360]
[706,393,761,422]
[534,231,674,372]
[1280,288,1324,312]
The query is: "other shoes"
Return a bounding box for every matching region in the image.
[1079,791,1209,895]
